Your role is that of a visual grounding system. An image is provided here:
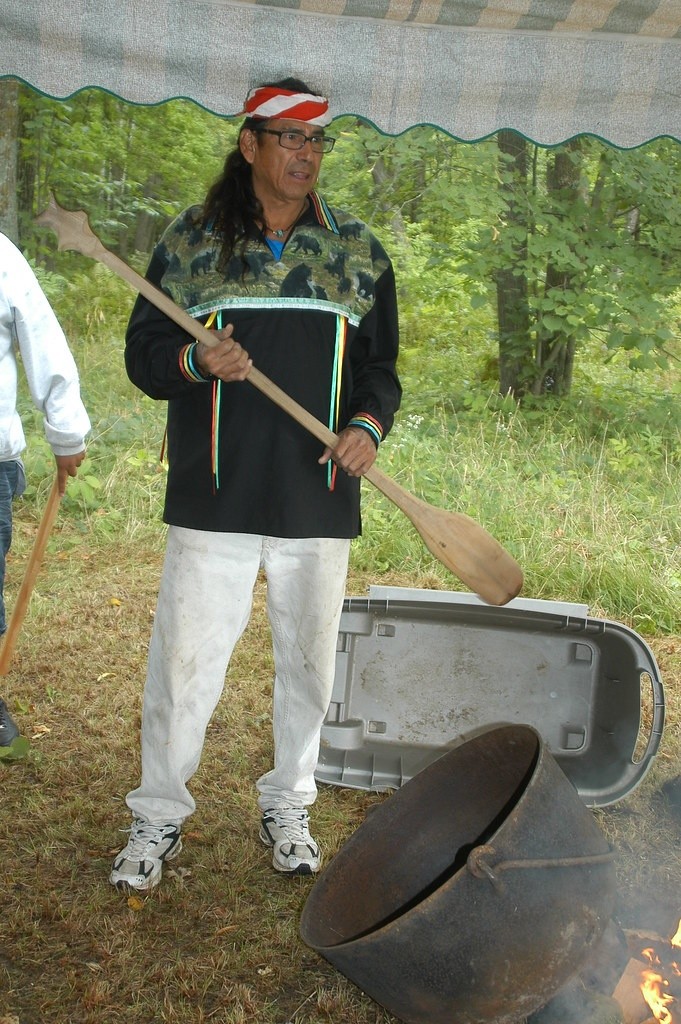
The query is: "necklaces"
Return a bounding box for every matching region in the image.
[267,203,305,237]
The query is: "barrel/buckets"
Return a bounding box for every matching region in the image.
[299,724,615,1024]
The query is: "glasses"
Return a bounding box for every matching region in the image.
[262,128,336,154]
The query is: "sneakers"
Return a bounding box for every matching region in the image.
[0,696,31,765]
[259,807,322,875]
[109,818,183,892]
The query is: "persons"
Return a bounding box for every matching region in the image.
[108,77,402,892]
[0,234,92,744]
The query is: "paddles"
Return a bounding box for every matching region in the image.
[5,479,69,688]
[49,206,529,607]
